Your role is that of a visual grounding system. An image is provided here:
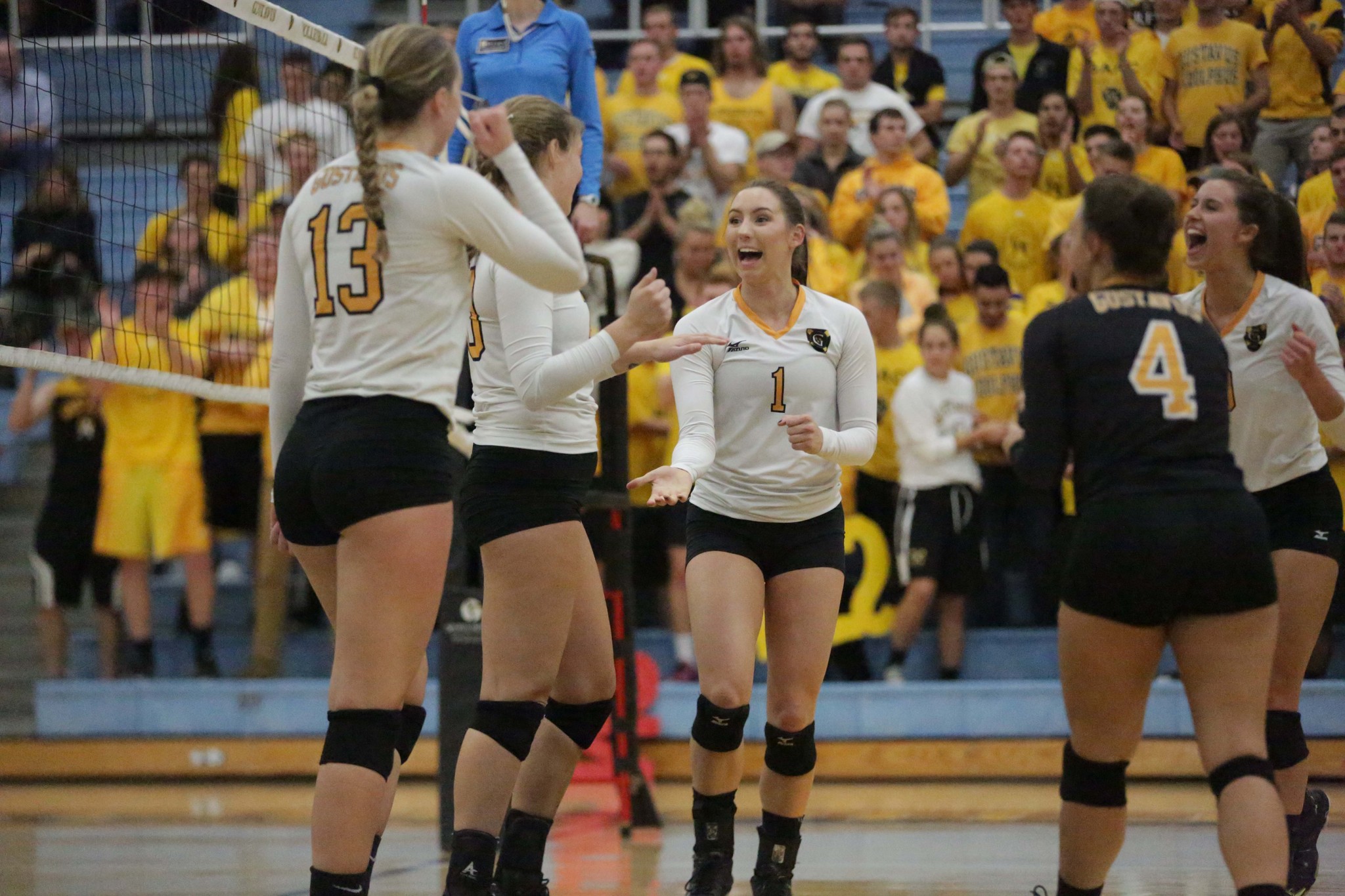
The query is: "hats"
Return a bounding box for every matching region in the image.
[754,130,798,157]
[134,261,185,287]
[981,52,1020,82]
[680,70,711,89]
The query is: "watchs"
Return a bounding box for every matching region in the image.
[578,193,600,208]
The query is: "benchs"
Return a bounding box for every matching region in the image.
[0,161,1345,745]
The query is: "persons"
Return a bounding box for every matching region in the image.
[0,1,1345,688]
[629,181,877,896]
[1000,173,1291,895]
[269,24,587,894]
[1179,163,1345,895]
[445,95,736,896]
